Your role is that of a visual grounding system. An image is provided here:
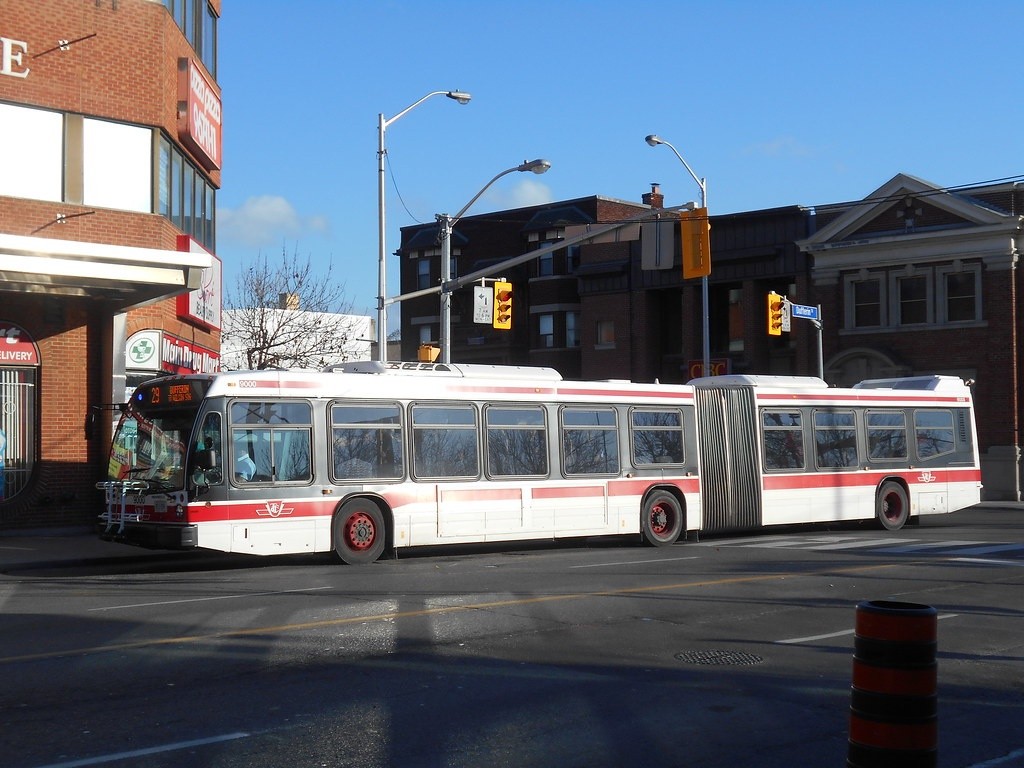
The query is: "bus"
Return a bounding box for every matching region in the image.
[82,358,983,568]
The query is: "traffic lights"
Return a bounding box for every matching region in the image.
[493,282,513,329]
[768,294,785,336]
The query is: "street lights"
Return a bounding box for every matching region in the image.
[377,88,471,364]
[435,159,552,367]
[644,135,710,379]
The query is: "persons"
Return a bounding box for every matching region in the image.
[234,442,257,483]
